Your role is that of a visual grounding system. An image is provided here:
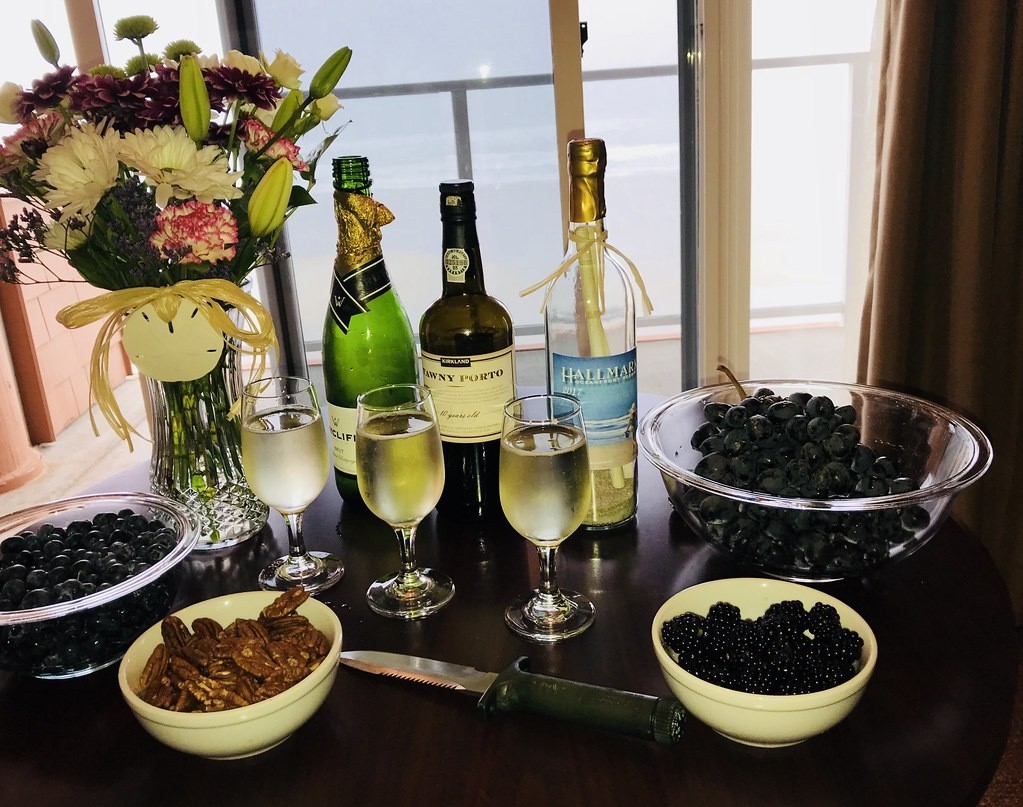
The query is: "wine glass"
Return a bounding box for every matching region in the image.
[499,393,597,640]
[240,376,344,597]
[356,383,456,620]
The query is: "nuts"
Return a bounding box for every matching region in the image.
[136,587,331,712]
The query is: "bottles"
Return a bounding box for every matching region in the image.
[321,151,417,510]
[418,178,518,525]
[544,137,639,533]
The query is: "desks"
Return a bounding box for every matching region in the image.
[0,384,1023,807]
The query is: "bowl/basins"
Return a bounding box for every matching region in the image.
[635,378,993,584]
[117,592,343,762]
[0,492,201,680]
[652,577,878,749]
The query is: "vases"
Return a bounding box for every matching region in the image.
[121,273,271,552]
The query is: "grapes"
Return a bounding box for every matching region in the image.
[0,509,179,675]
[680,385,931,578]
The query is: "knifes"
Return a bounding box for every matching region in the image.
[340,651,686,746]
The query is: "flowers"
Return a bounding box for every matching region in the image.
[0,7,369,491]
